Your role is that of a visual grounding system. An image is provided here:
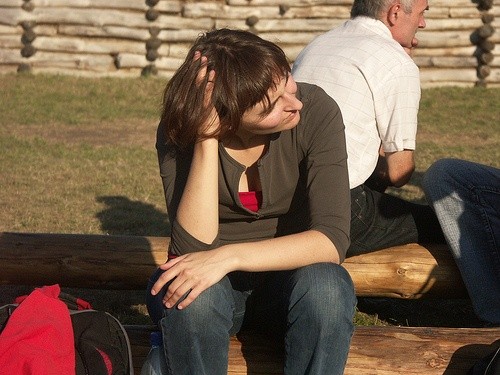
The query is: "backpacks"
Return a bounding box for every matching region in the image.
[0,284,135,375]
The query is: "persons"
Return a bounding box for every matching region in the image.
[292,0,445,260]
[151,28,356,375]
[423,158,500,375]
